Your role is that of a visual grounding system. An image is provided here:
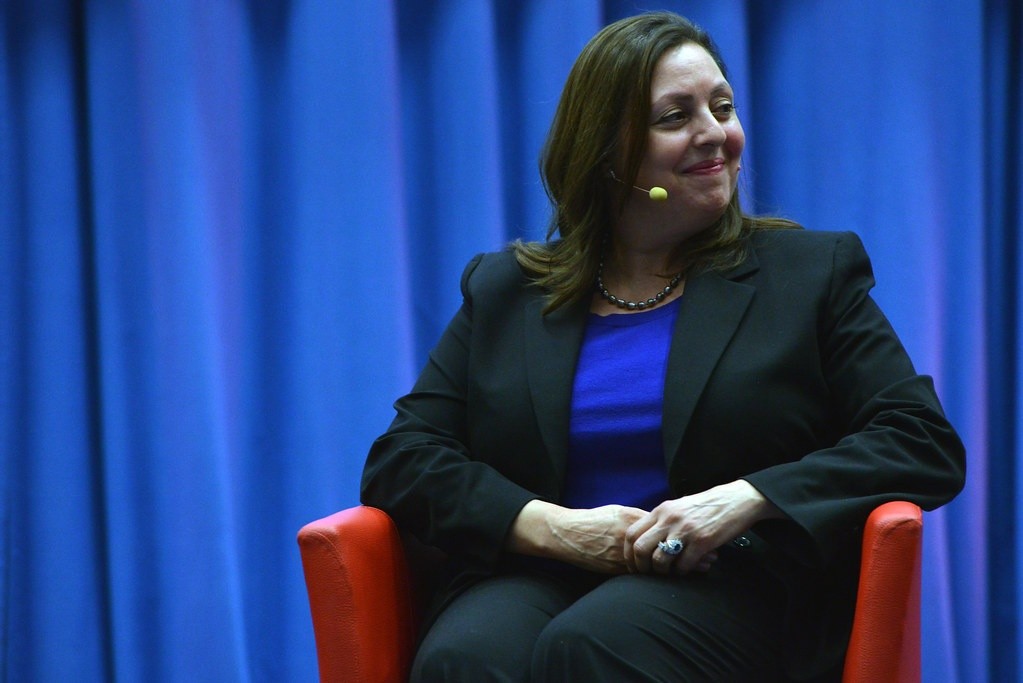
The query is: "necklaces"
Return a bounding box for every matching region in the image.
[592,237,684,310]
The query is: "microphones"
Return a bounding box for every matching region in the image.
[609,171,668,201]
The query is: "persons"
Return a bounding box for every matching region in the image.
[356,11,965,683]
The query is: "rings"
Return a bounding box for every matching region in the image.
[655,536,686,554]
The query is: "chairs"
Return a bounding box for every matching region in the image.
[299,501,924,683]
